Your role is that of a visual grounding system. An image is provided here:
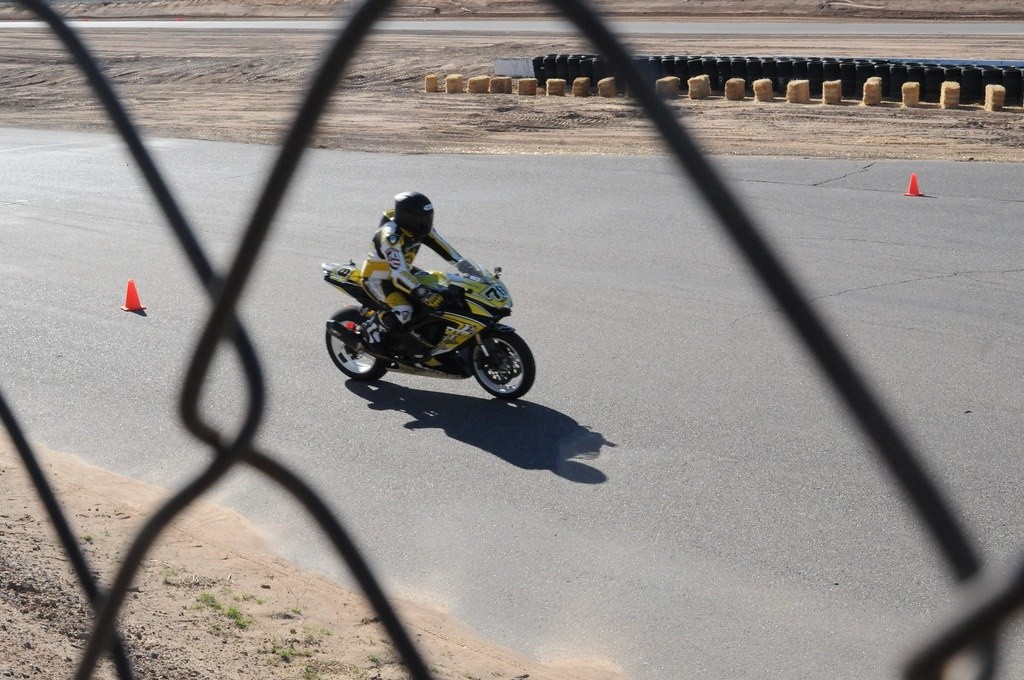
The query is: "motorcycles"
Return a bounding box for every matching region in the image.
[316,253,539,402]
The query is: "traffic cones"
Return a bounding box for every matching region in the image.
[903,172,925,197]
[120,278,147,311]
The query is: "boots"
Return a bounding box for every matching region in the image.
[362,304,391,352]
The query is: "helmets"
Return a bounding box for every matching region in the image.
[394,191,434,237]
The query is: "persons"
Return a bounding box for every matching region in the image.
[350,191,485,354]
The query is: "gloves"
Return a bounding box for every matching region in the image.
[458,260,484,279]
[411,285,445,313]
